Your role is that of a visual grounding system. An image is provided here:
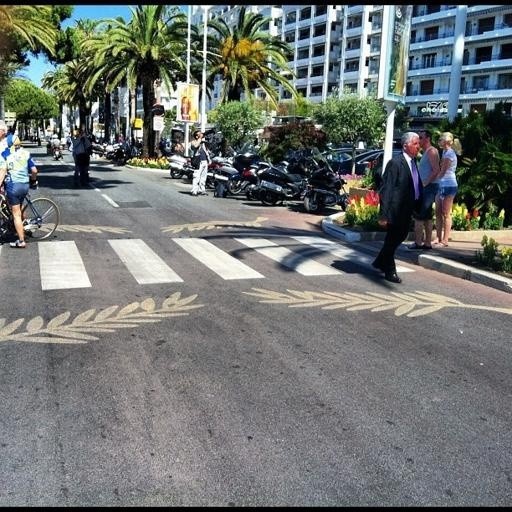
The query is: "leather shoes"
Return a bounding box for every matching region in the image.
[372,261,401,283]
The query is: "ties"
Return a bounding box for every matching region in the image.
[411,159,419,200]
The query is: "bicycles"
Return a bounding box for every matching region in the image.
[0,181,62,244]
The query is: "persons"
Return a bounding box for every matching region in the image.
[429,131,464,250]
[44,125,127,190]
[189,130,213,197]
[371,131,426,285]
[0,132,38,249]
[0,118,8,149]
[404,127,442,253]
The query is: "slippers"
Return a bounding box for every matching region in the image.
[407,239,448,250]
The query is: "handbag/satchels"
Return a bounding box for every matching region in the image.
[191,155,200,170]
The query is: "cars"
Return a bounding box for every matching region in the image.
[301,139,401,179]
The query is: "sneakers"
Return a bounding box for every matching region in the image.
[192,192,209,197]
[10,239,26,248]
